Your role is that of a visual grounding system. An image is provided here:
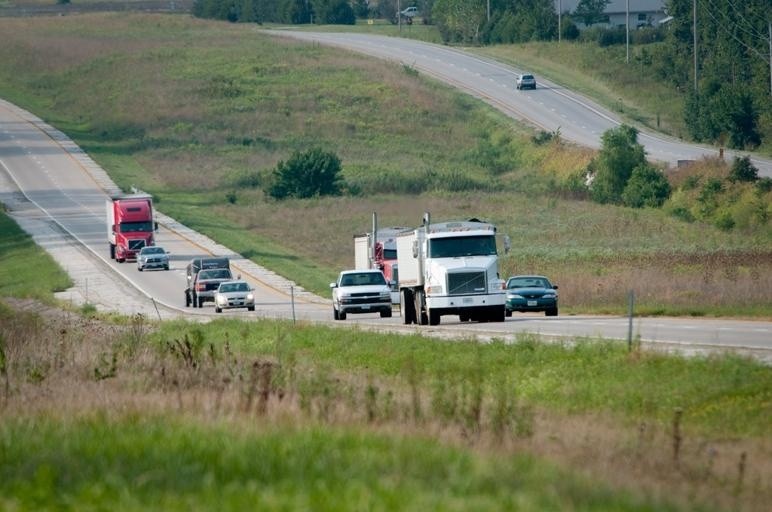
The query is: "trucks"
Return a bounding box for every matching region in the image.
[353,211,510,326]
[106,193,159,263]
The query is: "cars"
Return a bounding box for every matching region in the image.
[329,268,394,320]
[516,73,536,90]
[135,246,170,271]
[401,7,418,17]
[184,257,255,312]
[506,275,558,316]
